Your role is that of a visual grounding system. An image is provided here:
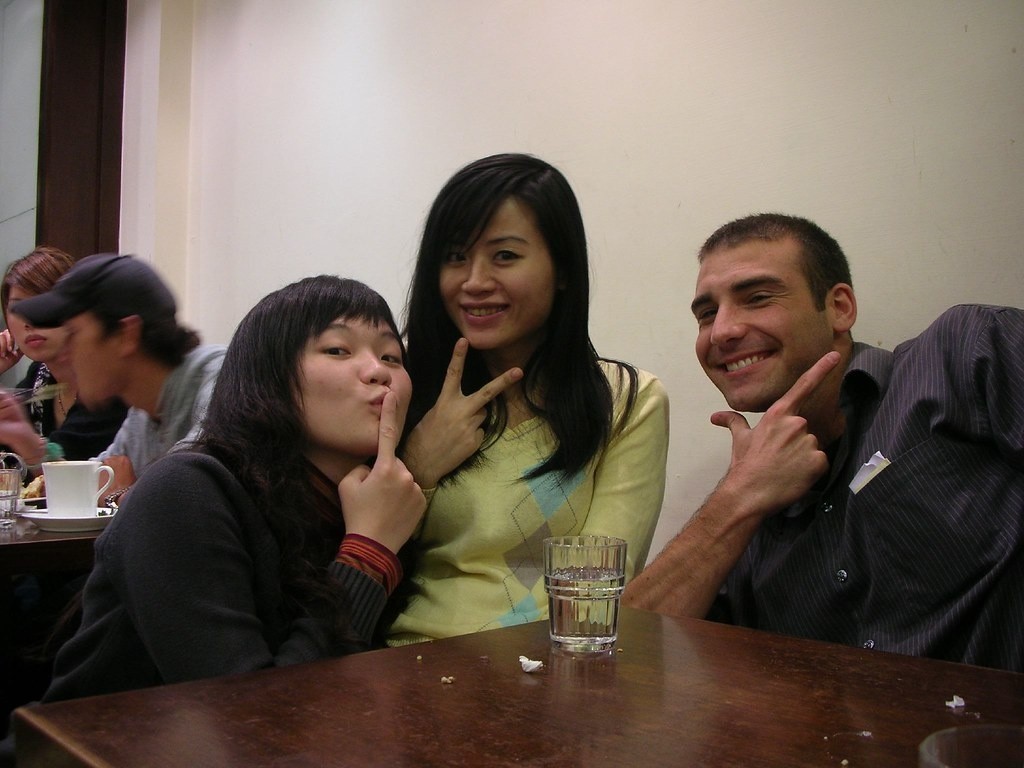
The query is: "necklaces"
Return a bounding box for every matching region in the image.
[58,390,76,417]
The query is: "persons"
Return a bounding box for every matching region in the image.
[1,246,227,513]
[36,275,428,706]
[620,213,1024,671]
[383,155,669,647]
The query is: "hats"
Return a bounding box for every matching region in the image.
[10,253,175,329]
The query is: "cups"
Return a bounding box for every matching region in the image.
[41,461,114,516]
[918,724,1024,768]
[0,469,21,526]
[542,535,628,652]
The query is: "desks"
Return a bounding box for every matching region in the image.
[0,508,104,576]
[12,610,1023,768]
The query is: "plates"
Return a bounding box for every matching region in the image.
[22,508,118,531]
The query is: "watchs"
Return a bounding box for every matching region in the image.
[105,486,130,508]
